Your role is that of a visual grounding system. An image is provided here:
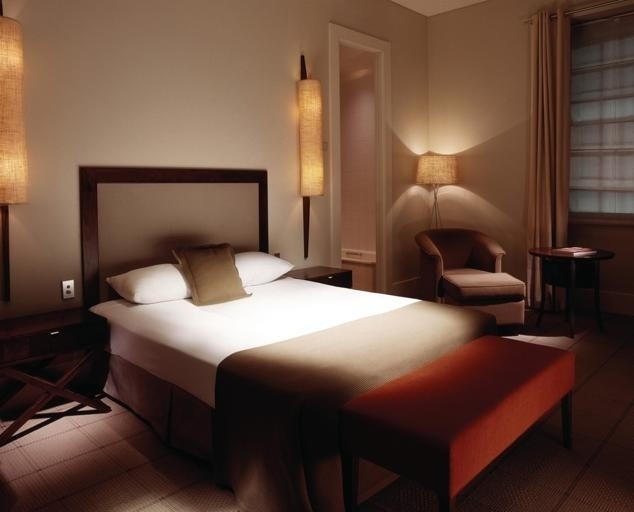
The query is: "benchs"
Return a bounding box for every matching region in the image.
[342,335,576,512]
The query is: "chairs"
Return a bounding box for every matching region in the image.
[414,226,526,329]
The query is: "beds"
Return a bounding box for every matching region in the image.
[80,165,500,512]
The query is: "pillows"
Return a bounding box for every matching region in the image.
[106,263,191,306]
[233,250,294,291]
[173,241,252,306]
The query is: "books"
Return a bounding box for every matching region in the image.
[553,246,596,255]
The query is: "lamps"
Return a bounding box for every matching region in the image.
[298,55,324,258]
[0,0,31,305]
[415,155,458,230]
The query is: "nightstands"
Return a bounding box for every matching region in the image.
[283,265,350,288]
[0,307,112,445]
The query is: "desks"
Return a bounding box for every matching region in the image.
[531,246,614,335]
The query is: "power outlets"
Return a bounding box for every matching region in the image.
[61,279,75,300]
